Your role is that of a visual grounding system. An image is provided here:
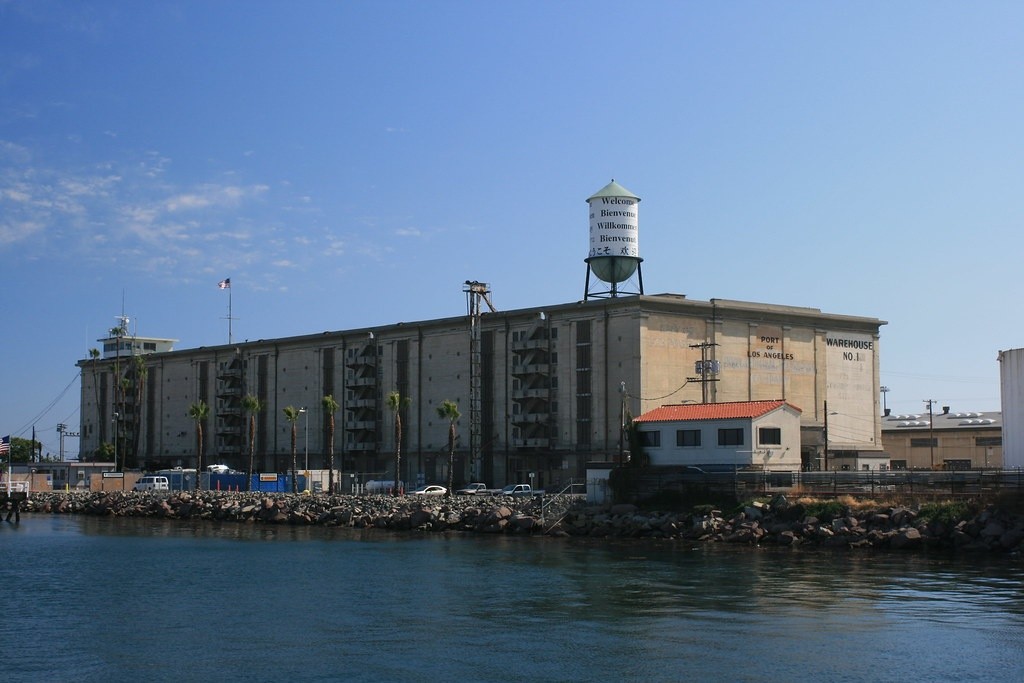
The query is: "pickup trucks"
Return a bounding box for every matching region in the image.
[493,484,546,499]
[457,482,503,495]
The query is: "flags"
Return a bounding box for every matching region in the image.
[218,278,230,288]
[0,435,9,452]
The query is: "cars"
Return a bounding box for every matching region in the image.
[406,485,448,499]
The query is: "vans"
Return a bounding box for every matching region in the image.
[133,476,169,491]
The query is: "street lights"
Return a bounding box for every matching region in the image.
[824,412,839,471]
[298,407,308,472]
[114,412,119,472]
